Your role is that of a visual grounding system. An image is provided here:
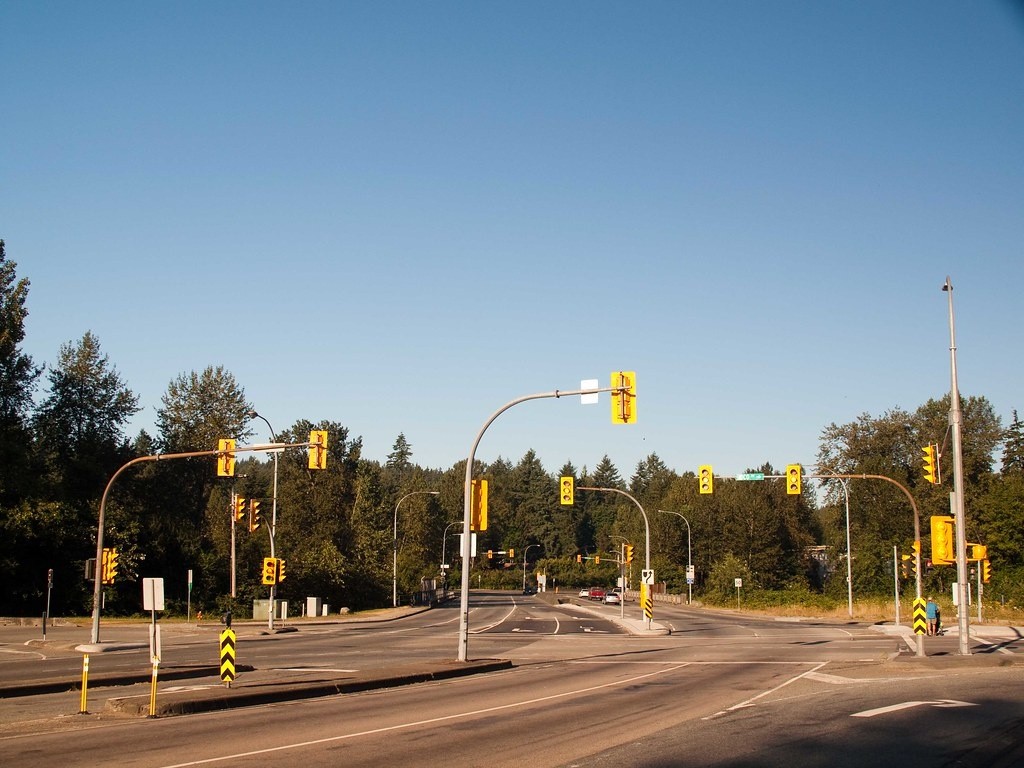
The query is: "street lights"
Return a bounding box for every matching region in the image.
[523,544,541,591]
[608,535,632,589]
[657,510,692,605]
[942,275,972,656]
[443,521,464,592]
[392,491,440,607]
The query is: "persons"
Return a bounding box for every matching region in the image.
[926,597,937,636]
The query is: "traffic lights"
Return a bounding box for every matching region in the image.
[251,499,261,532]
[263,558,276,584]
[103,548,121,583]
[910,543,918,576]
[560,477,574,505]
[902,554,909,579]
[920,442,935,483]
[984,559,992,583]
[279,560,287,582]
[698,466,713,495]
[627,546,634,562]
[234,495,246,522]
[787,464,801,495]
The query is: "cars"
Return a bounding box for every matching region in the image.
[522,586,537,595]
[578,588,628,605]
